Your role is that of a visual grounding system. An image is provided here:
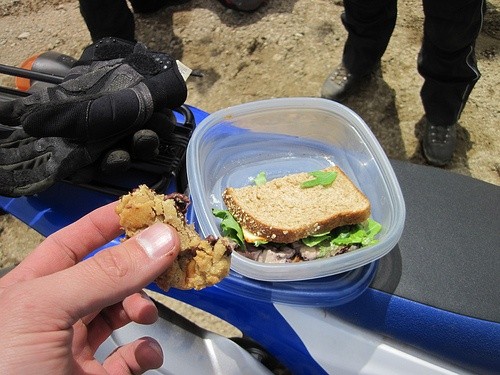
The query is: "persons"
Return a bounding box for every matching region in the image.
[320,0,484,166]
[79,0,191,44]
[0,193,181,375]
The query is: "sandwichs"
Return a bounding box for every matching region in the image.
[212,165,383,264]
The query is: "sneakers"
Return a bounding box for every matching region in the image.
[321,58,361,100]
[414,114,455,165]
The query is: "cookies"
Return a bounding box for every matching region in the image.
[116,184,232,290]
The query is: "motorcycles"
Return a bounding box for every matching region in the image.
[0,49,500,374]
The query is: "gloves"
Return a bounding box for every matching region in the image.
[0,37,190,197]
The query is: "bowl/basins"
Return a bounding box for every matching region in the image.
[186,95,406,280]
